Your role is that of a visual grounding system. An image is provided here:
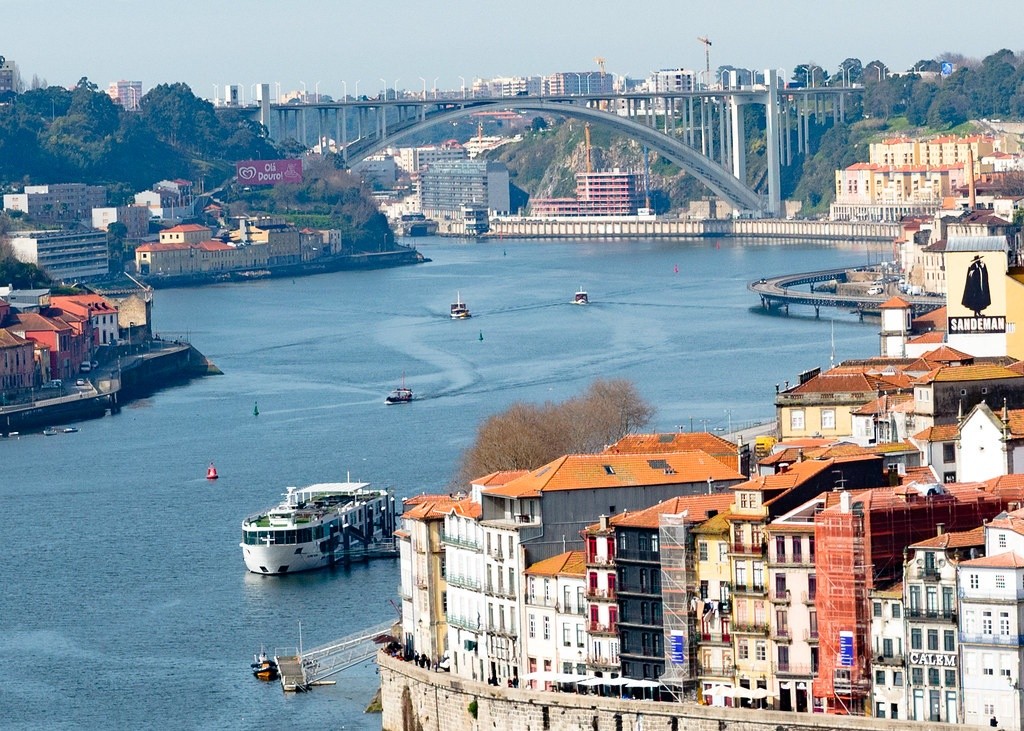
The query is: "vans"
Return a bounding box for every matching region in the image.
[81,361,90,372]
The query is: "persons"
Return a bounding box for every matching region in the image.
[486,673,519,687]
[387,642,439,673]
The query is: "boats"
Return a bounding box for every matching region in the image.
[383,371,414,405]
[239,472,392,576]
[575,287,587,305]
[452,290,467,320]
[252,643,276,682]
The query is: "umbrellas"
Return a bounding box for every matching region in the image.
[722,685,752,708]
[740,686,780,709]
[371,634,390,650]
[374,635,397,651]
[521,670,663,702]
[701,684,733,708]
